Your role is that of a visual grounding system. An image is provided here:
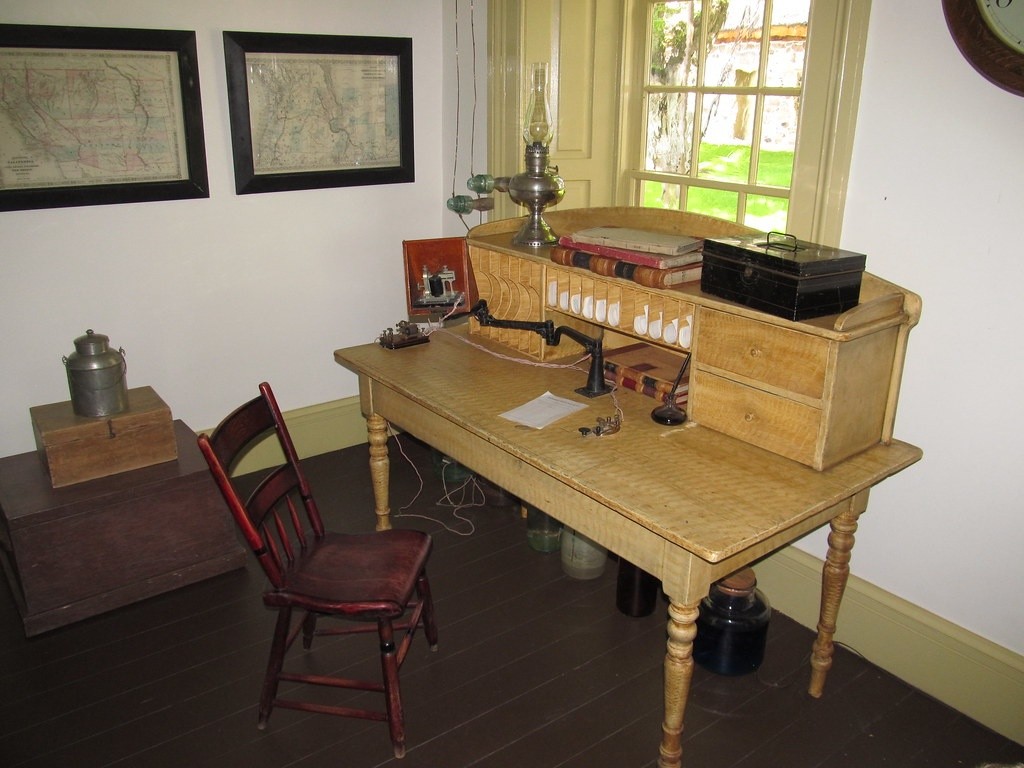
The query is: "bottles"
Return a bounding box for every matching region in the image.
[615,556,655,616]
[482,476,519,507]
[560,524,606,579]
[691,567,771,677]
[62,329,130,416]
[527,502,563,552]
[432,446,470,481]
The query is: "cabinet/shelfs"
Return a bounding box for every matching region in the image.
[465,206,923,472]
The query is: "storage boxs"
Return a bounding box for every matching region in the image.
[28,385,181,489]
[700,230,867,324]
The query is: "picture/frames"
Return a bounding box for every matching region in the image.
[0,23,211,212]
[221,30,416,194]
[401,236,471,315]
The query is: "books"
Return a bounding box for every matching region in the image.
[551,226,703,288]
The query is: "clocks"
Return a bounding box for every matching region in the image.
[941,0,1024,98]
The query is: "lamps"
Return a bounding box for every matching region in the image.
[510,61,567,247]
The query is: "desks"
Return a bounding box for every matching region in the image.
[334,323,923,768]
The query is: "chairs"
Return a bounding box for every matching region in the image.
[196,381,440,760]
